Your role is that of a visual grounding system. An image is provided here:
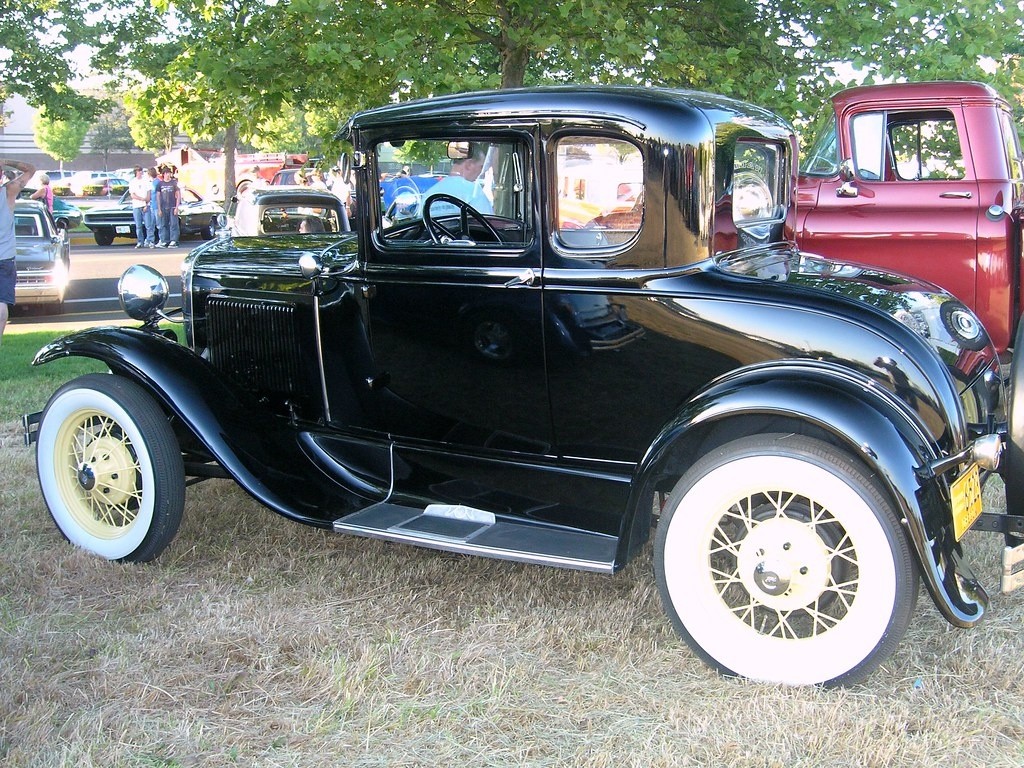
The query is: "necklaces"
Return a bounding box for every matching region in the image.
[448,171,462,176]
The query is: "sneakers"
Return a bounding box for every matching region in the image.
[134,238,180,249]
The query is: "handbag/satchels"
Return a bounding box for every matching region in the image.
[36,197,50,209]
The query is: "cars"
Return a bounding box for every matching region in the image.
[14,200,70,314]
[271,169,358,231]
[33,170,130,186]
[229,189,351,237]
[84,185,227,246]
[23,86,1024,690]
[583,81,1023,379]
[16,188,83,230]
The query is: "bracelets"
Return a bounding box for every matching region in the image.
[346,206,350,208]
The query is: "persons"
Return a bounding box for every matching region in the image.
[417,145,497,220]
[0,158,35,336]
[30,173,53,214]
[293,166,352,233]
[129,165,181,249]
